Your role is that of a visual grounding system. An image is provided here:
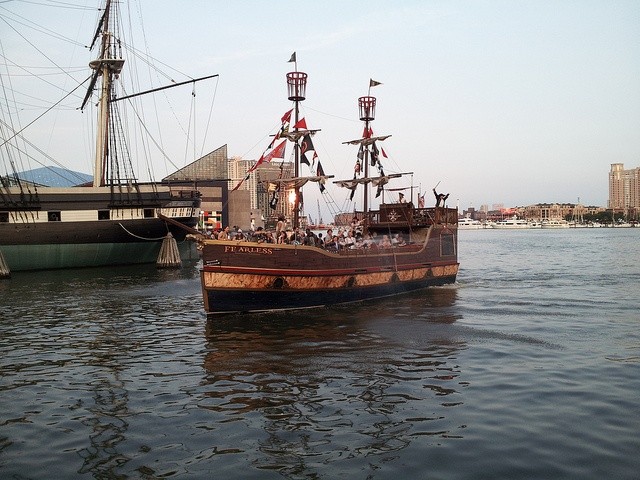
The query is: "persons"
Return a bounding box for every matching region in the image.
[432,188,449,207]
[209,214,407,248]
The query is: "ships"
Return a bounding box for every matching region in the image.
[457,217,493,230]
[1,1,202,271]
[541,219,570,229]
[570,219,640,228]
[490,215,541,229]
[185,52,460,318]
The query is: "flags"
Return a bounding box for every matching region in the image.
[349,172,357,201]
[370,142,379,166]
[316,161,326,193]
[374,169,385,197]
[357,143,365,173]
[299,134,315,167]
[262,139,286,164]
